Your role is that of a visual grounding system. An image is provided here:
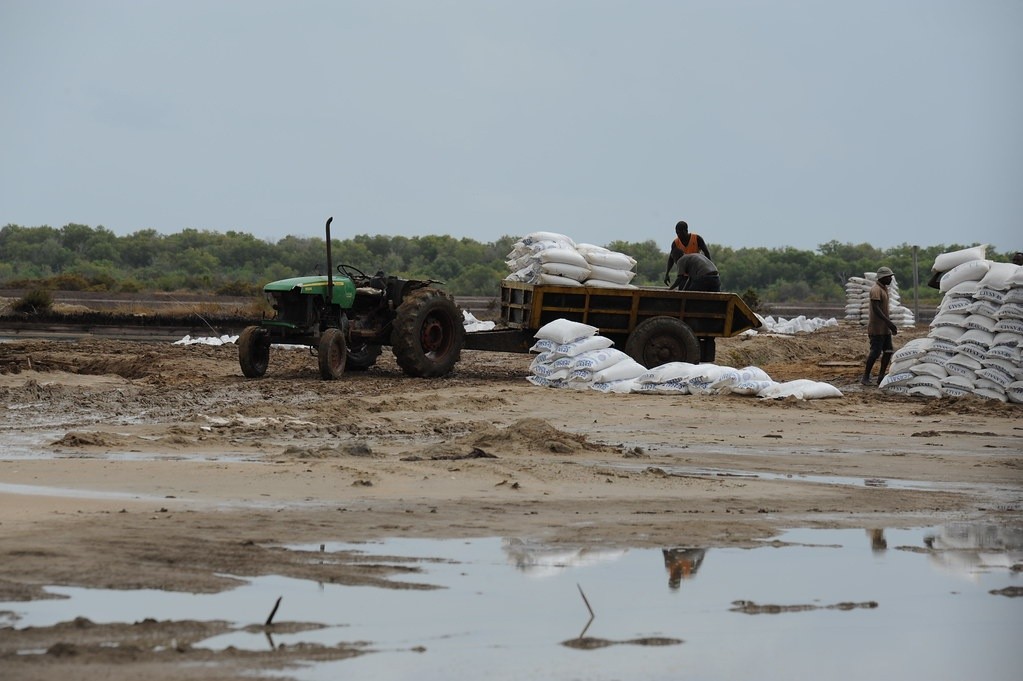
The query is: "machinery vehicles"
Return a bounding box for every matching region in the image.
[238,215,465,380]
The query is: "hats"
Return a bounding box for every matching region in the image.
[875,266,895,279]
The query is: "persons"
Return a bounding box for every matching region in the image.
[860,267,897,385]
[664,221,711,290]
[667,248,721,292]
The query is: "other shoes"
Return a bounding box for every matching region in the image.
[860,378,877,387]
